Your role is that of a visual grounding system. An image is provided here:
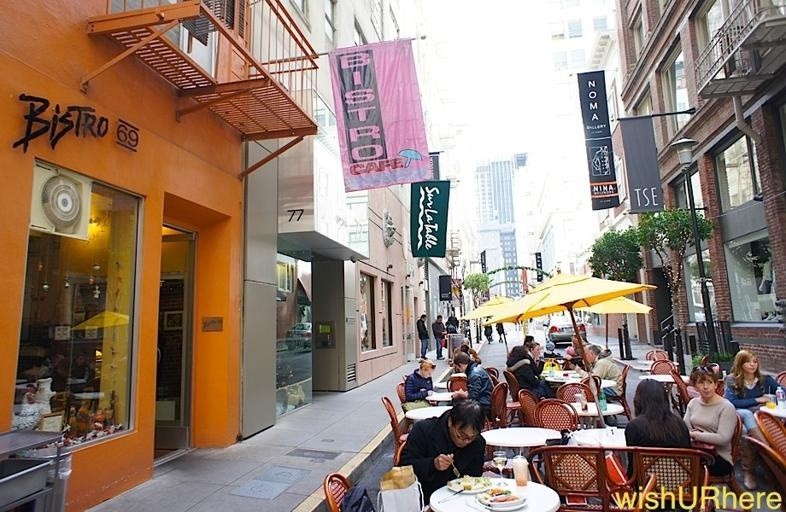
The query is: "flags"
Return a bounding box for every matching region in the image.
[328,38,433,191]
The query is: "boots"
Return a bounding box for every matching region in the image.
[737,441,758,490]
[747,426,771,449]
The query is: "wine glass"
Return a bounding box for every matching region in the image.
[492,451,508,487]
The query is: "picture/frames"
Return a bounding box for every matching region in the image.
[161,308,183,333]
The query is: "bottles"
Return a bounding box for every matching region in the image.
[776,386,786,414]
[580,389,588,411]
[599,390,607,412]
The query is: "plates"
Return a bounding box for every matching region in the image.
[476,495,528,512]
[485,494,525,507]
[447,477,491,494]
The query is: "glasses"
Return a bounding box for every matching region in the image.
[692,365,714,374]
[452,422,477,440]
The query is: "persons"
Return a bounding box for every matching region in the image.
[452,352,494,418]
[562,333,588,372]
[684,366,737,476]
[46,353,91,392]
[417,314,429,359]
[527,342,545,375]
[404,359,436,414]
[448,311,459,331]
[461,345,471,356]
[396,399,486,510]
[759,257,778,320]
[453,338,481,365]
[495,322,504,343]
[523,335,535,347]
[445,321,457,334]
[624,379,692,512]
[484,320,492,344]
[506,345,555,399]
[432,315,447,360]
[576,345,622,426]
[726,350,786,491]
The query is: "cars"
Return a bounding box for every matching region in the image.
[543,316,590,344]
[283,321,313,352]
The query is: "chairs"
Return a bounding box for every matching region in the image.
[39,411,65,433]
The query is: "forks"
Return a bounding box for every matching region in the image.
[448,455,461,478]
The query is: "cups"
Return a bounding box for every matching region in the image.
[575,394,581,407]
[763,394,776,410]
[512,455,529,486]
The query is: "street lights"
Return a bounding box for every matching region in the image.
[670,136,722,364]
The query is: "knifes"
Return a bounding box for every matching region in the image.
[438,490,463,503]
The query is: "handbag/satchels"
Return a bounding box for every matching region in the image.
[376,473,425,511]
[688,439,717,455]
[402,400,431,411]
[341,486,375,512]
